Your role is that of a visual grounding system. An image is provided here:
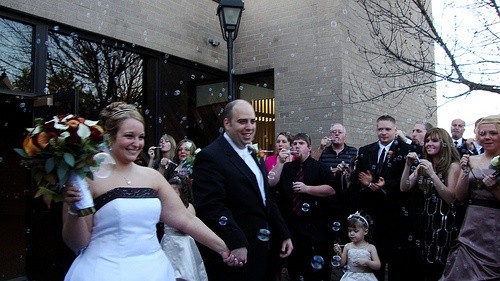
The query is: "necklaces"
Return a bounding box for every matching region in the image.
[114,164,134,185]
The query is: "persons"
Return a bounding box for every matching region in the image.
[147,98,500,281]
[60,102,247,281]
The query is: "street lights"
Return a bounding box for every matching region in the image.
[212,0,245,103]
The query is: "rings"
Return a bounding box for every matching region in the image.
[299,187,301,190]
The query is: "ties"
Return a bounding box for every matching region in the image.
[376,149,386,177]
[293,164,304,214]
[454,141,458,146]
